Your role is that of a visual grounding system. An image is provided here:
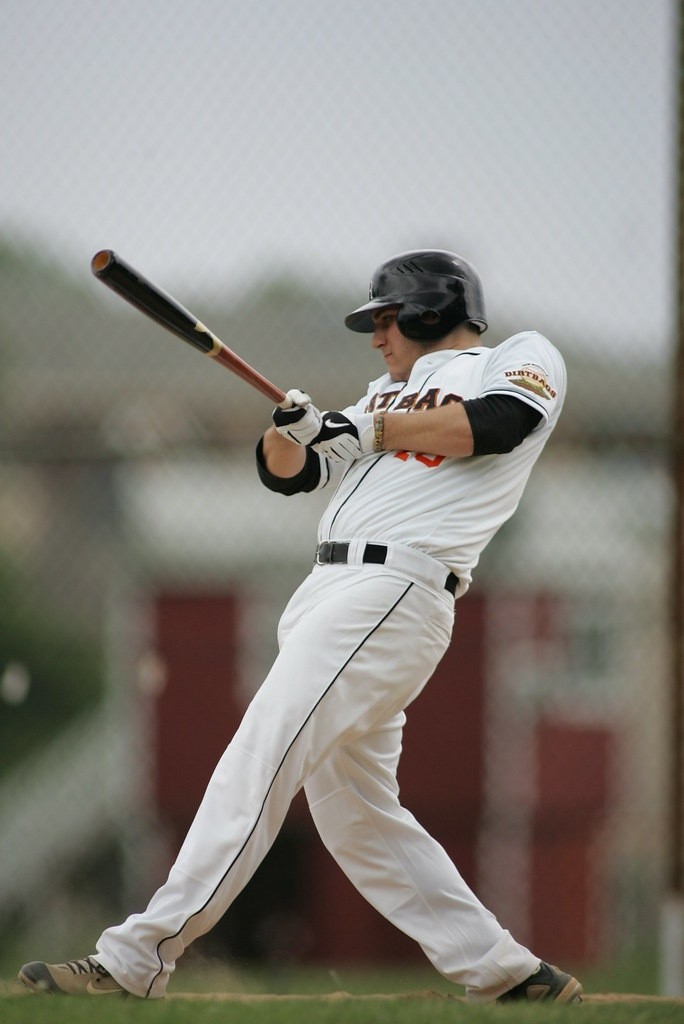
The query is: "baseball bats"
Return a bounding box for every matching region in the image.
[90,247,297,418]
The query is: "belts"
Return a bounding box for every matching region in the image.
[314,540,459,598]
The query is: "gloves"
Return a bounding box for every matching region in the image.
[308,410,376,463]
[272,389,323,447]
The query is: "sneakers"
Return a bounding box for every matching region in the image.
[17,956,137,999]
[495,960,585,1007]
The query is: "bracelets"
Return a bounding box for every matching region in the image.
[373,411,388,452]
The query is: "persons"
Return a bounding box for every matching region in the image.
[18,249,584,1008]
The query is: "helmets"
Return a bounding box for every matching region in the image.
[344,250,489,342]
